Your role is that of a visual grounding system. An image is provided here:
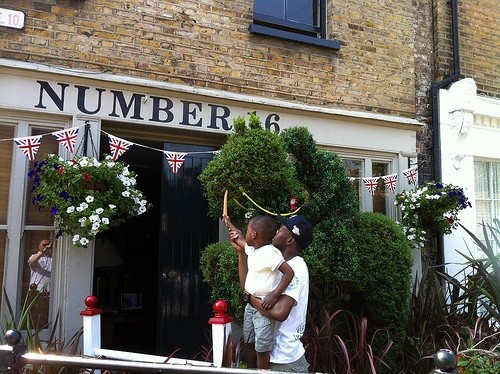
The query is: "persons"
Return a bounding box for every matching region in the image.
[222,214,294,369]
[228,215,313,373]
[26,239,53,314]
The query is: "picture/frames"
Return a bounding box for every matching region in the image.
[118,289,143,311]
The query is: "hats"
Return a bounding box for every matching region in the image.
[278,213,314,250]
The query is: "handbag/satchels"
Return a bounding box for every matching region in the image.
[30,283,37,292]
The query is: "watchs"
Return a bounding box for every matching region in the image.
[242,292,251,302]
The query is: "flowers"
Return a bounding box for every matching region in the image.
[393,179,473,250]
[26,151,154,249]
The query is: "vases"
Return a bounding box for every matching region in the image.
[414,202,442,227]
[85,182,110,193]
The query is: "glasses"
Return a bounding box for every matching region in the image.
[40,243,49,246]
